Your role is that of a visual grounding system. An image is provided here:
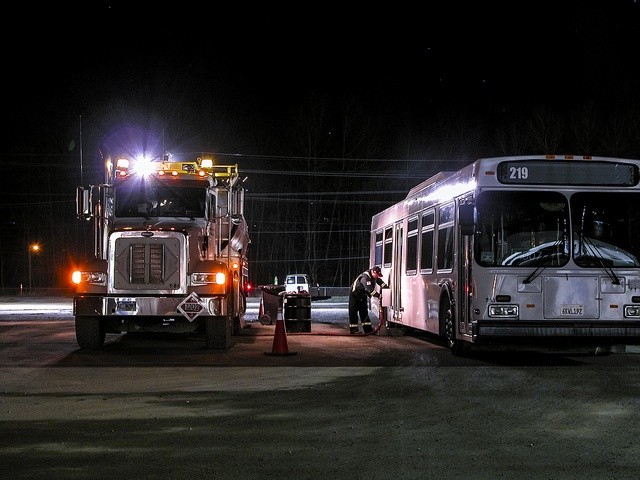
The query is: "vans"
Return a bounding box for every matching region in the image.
[283,273,311,295]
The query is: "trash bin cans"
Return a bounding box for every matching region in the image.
[259,285,285,325]
[284,294,311,333]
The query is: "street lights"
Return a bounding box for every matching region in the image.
[28,244,39,295]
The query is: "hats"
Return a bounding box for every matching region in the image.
[373,266,384,277]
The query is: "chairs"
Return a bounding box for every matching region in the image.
[481,251,494,263]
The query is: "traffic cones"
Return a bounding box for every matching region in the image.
[256,298,264,321]
[263,308,297,357]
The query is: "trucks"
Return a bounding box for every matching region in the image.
[71,157,254,351]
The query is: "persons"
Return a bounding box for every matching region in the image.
[349,266,390,335]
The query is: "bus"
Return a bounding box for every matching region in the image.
[368,155,640,357]
